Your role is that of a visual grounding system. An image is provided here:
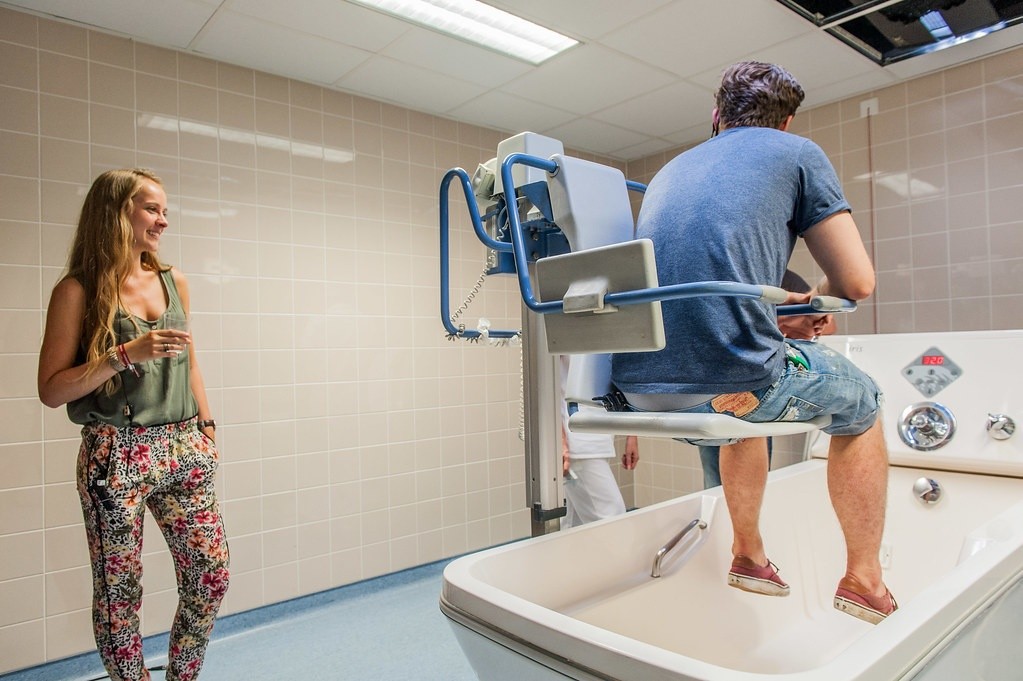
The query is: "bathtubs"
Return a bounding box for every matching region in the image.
[438,328,1023,681]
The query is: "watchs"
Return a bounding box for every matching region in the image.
[199,420,216,430]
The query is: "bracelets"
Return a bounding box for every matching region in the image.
[108,347,126,372]
[119,344,131,367]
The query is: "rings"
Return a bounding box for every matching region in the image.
[163,344,169,352]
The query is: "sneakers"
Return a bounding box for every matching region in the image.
[727,556,791,596]
[834,577,899,624]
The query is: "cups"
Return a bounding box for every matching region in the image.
[164,313,192,354]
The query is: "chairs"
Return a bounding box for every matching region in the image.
[534,238,858,441]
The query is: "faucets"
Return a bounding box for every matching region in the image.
[913,475,940,504]
[985,414,1016,440]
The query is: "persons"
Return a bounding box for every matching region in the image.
[38,167,231,681]
[699,435,772,489]
[611,59,898,626]
[559,401,639,532]
